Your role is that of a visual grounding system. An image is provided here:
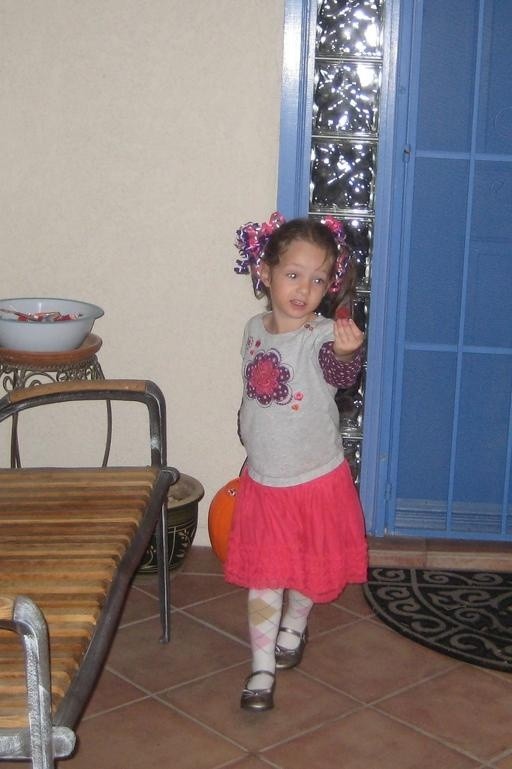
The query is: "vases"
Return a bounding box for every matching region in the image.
[135,473,204,575]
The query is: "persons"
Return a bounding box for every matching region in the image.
[224,211,369,711]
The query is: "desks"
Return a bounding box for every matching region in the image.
[0,332,112,469]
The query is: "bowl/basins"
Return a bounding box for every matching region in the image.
[0,297,105,354]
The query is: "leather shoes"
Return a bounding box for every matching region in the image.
[276,622,307,670]
[238,668,277,712]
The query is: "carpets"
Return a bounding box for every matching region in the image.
[360,568,511,674]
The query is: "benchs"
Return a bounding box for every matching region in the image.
[0,377,181,769]
